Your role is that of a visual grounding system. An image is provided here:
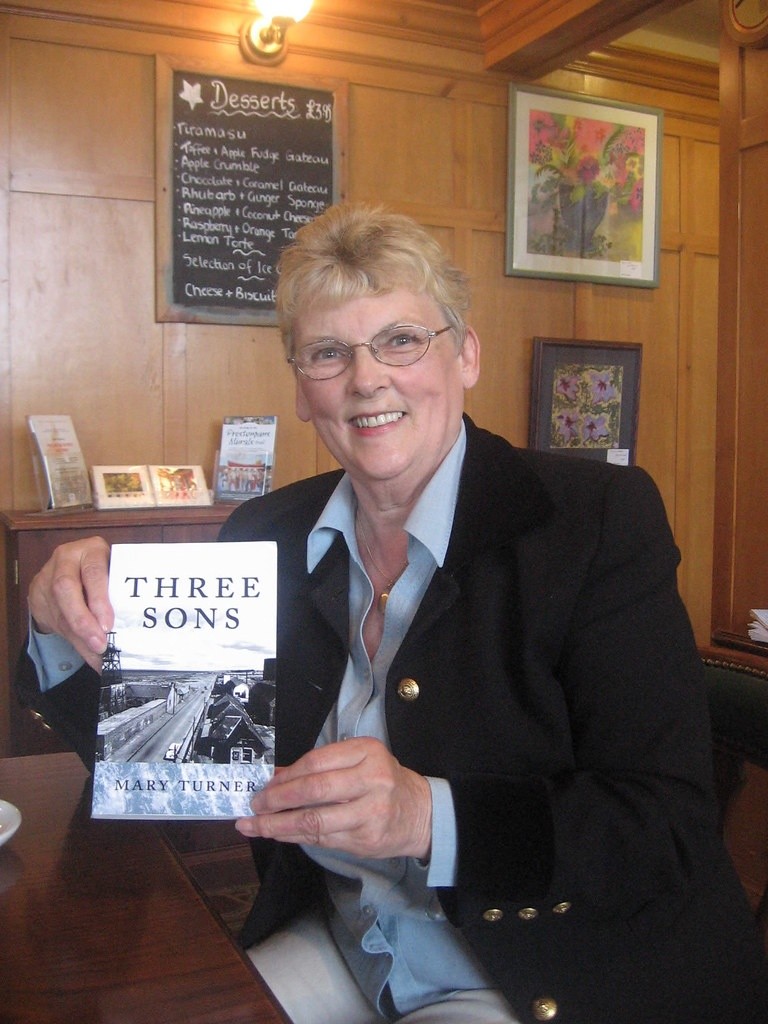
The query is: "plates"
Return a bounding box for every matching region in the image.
[0,799,21,844]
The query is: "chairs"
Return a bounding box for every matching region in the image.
[249,649,768,927]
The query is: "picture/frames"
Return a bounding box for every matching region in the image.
[503,80,664,289]
[527,336,643,465]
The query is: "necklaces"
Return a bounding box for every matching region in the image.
[356,505,406,613]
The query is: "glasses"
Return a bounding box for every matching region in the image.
[286,325,452,380]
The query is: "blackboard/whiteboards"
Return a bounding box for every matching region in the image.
[153,49,351,328]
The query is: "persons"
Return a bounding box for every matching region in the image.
[14,198,768,1024]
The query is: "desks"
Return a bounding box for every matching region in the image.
[0,751,292,1024]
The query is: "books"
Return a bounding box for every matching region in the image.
[89,540,277,823]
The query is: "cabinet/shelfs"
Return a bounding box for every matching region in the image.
[0,502,237,758]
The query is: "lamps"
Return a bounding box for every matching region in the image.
[239,0,311,67]
